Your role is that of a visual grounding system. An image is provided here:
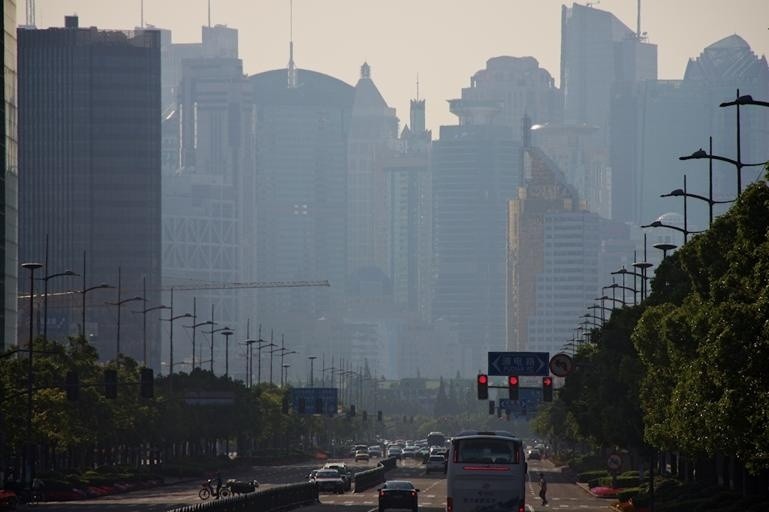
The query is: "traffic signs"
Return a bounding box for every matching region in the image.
[486,351,550,376]
[498,398,528,410]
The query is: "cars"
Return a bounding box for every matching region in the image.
[524,438,544,460]
[0,478,164,512]
[305,431,452,492]
[374,480,421,512]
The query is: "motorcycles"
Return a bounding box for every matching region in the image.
[198,476,233,500]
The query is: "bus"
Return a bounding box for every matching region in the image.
[444,428,528,512]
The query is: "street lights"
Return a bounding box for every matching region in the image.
[76,281,113,338]
[19,260,46,486]
[131,305,173,362]
[658,185,738,228]
[101,296,149,355]
[640,213,705,247]
[162,309,378,417]
[709,92,769,112]
[558,229,677,356]
[673,147,767,196]
[34,270,74,354]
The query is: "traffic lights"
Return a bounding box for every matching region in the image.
[507,376,519,400]
[542,377,553,402]
[476,373,488,401]
[487,400,495,415]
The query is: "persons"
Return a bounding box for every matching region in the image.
[32,474,47,501]
[215,468,222,500]
[538,472,548,508]
